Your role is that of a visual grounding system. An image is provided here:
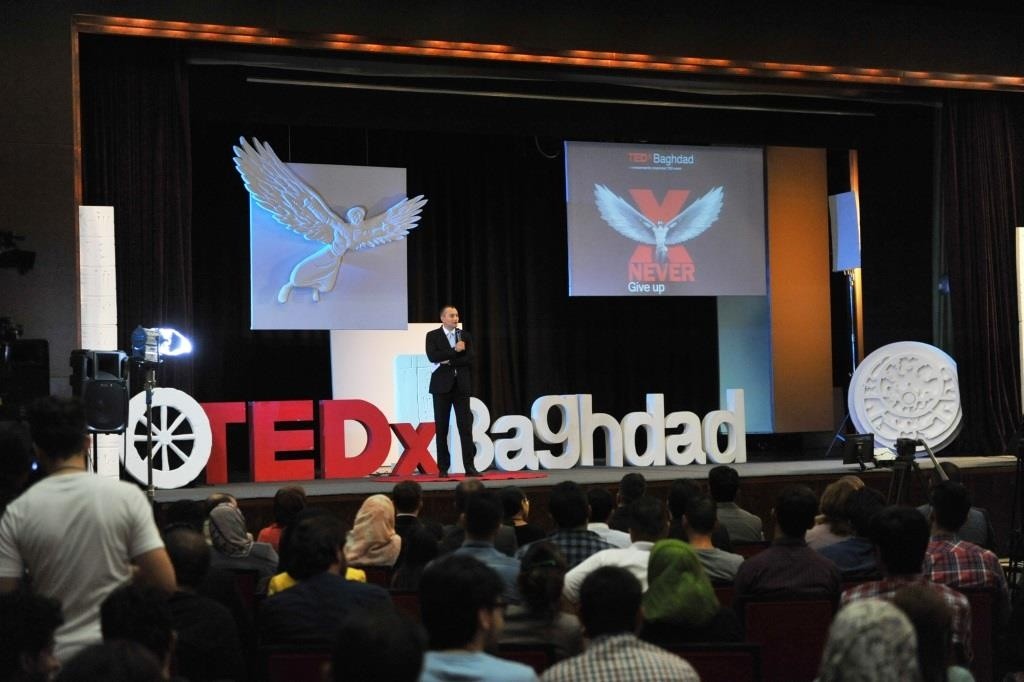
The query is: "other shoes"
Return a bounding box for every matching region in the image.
[464,464,478,474]
[439,470,447,477]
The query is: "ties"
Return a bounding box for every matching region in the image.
[448,332,455,347]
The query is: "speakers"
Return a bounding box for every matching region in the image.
[69,349,128,435]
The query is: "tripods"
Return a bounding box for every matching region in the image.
[884,462,933,509]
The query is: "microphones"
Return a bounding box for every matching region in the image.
[456,328,462,342]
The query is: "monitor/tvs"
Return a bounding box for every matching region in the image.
[843,433,874,471]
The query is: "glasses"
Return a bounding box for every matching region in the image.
[475,602,507,616]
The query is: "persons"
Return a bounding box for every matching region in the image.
[634,216,682,274]
[275,206,395,304]
[425,306,482,478]
[0,396,1024,682]
